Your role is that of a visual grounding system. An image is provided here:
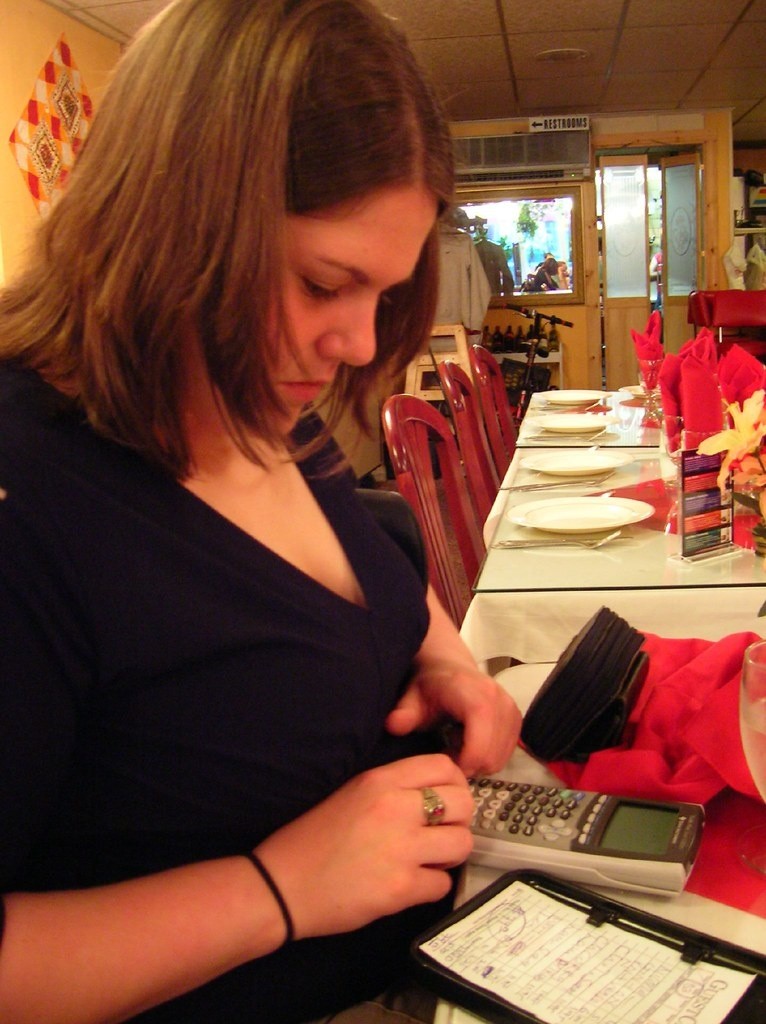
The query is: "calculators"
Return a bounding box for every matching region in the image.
[452,775,707,899]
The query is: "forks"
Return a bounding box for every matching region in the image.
[491,528,620,549]
[517,470,620,491]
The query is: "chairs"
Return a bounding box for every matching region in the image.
[688,290,766,358]
[381,395,485,632]
[467,344,520,482]
[436,359,500,527]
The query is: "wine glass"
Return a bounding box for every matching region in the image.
[638,356,665,424]
[657,413,684,535]
[737,639,766,875]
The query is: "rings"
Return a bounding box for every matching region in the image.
[420,785,447,824]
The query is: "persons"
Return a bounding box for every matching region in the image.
[650,252,663,313]
[530,252,569,290]
[0,0,521,1024]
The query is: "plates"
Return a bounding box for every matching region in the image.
[526,414,621,432]
[518,449,636,476]
[503,496,655,535]
[538,389,612,405]
[618,385,660,398]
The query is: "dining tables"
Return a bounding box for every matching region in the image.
[433,390,766,1024]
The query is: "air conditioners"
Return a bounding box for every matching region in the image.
[446,128,594,187]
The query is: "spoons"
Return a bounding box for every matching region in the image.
[585,443,602,450]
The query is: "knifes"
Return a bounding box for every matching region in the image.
[502,536,637,546]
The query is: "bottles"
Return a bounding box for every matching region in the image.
[482,321,560,355]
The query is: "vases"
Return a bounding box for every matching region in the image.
[752,523,766,558]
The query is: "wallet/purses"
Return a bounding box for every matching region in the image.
[520,605,648,763]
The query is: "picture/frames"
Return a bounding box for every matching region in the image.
[436,184,587,308]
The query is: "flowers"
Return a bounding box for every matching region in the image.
[696,389,766,520]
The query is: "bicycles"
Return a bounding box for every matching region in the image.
[503,302,574,422]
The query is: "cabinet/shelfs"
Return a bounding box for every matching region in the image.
[731,172,766,290]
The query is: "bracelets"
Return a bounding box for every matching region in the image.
[247,852,294,949]
